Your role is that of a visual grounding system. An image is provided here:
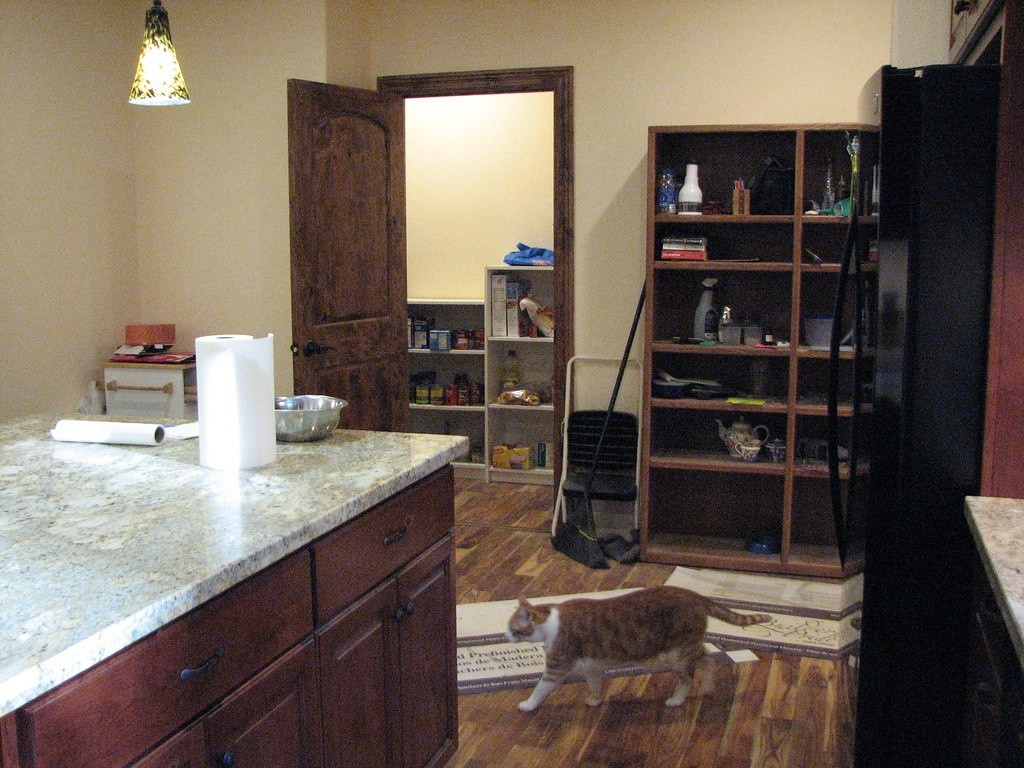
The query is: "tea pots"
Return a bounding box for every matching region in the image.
[715,415,770,457]
[734,435,764,462]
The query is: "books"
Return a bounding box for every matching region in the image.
[661,237,708,260]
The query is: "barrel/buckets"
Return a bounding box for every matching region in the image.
[414,380,446,406]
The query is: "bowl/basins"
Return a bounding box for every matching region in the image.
[804,316,834,348]
[274,395,348,441]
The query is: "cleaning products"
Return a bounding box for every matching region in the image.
[693,277,720,342]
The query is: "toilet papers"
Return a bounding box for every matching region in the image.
[195,333,277,470]
[50,419,167,446]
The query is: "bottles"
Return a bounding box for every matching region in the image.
[824,157,836,210]
[656,163,706,215]
[499,349,521,393]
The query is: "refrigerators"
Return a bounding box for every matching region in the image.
[827,58,999,767]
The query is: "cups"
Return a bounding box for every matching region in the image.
[733,189,750,214]
[718,323,766,347]
[765,437,785,462]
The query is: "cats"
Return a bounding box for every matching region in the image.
[505,585,773,714]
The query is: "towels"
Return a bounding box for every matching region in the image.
[164,422,200,440]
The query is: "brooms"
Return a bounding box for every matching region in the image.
[548,278,647,571]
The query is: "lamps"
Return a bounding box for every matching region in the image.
[128,0,191,107]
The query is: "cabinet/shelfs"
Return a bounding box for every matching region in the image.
[102,361,197,417]
[407,265,555,487]
[958,543,1024,768]
[0,412,469,768]
[639,124,880,580]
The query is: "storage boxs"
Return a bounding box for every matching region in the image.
[505,280,534,338]
[431,329,452,350]
[490,272,517,336]
